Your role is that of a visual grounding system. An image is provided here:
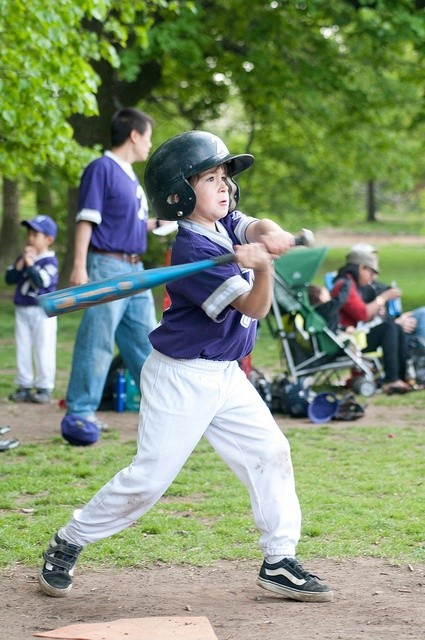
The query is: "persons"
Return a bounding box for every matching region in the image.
[350,241,419,391]
[306,270,357,340]
[5,214,59,403]
[60,107,171,445]
[38,130,334,601]
[327,250,412,398]
[404,305,425,386]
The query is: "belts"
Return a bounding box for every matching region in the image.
[90,248,141,265]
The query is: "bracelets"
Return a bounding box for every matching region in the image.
[156,220,160,227]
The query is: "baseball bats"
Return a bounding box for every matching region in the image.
[36,228,315,316]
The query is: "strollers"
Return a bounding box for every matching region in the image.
[269,235,387,424]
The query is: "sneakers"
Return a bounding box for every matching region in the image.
[256,556,334,602]
[29,391,52,403]
[9,388,30,401]
[38,531,83,597]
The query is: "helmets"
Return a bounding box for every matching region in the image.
[144,130,256,221]
[309,391,338,421]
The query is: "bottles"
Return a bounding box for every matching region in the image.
[113,369,125,412]
[386,281,404,316]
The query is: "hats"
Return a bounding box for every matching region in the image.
[20,215,58,240]
[346,244,380,275]
